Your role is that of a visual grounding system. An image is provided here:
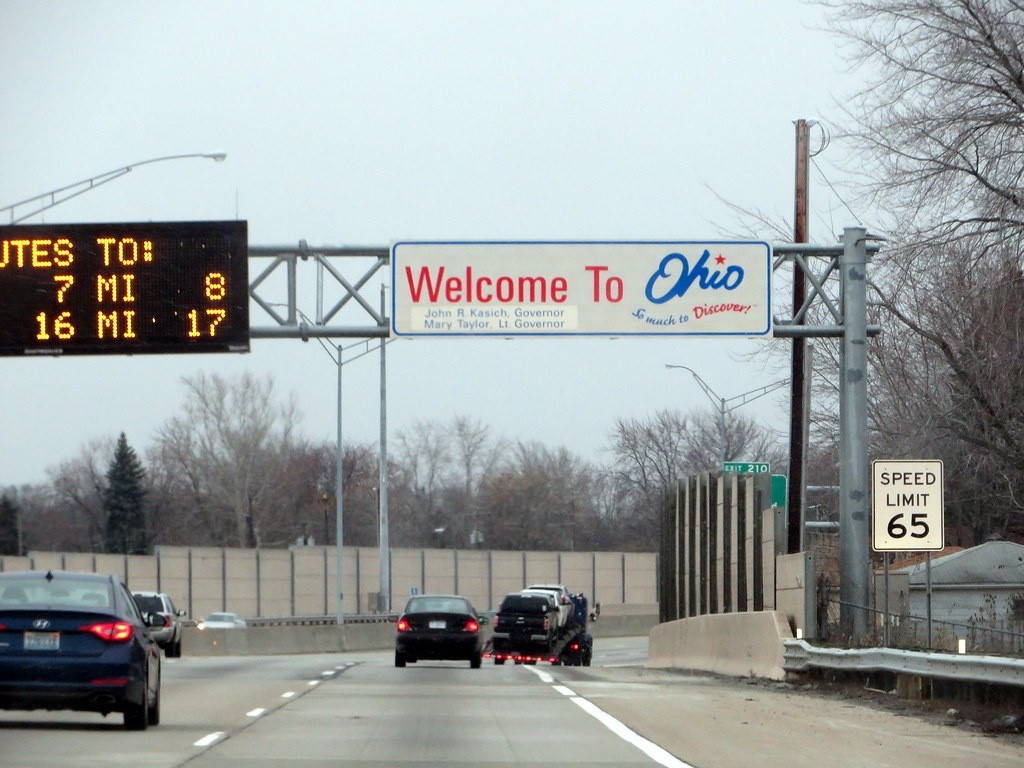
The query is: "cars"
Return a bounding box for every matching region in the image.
[0,571,169,732]
[197,612,248,632]
[387,595,490,669]
[480,584,575,654]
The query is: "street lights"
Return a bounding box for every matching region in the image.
[664,362,793,470]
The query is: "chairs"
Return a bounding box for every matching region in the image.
[3,587,29,605]
[81,594,106,606]
[416,603,427,611]
[449,603,461,612]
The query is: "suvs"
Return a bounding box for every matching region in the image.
[133,591,188,657]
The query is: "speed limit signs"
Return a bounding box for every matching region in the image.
[870,456,946,554]
